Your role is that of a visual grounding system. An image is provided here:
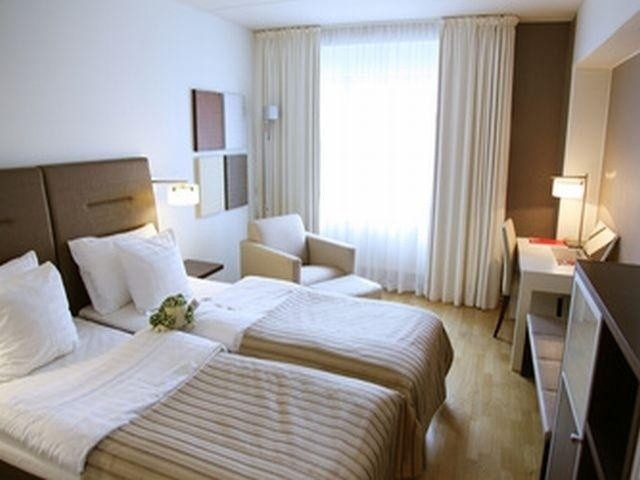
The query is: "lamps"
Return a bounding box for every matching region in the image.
[150,177,201,254]
[261,104,279,140]
[549,172,589,248]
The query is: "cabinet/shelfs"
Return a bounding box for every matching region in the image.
[542,258,640,479]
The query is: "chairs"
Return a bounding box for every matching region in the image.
[491,218,566,338]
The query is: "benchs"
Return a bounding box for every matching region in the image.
[518,310,568,480]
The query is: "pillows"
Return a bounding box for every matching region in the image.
[65,221,159,315]
[0,247,41,281]
[0,258,85,385]
[110,229,196,315]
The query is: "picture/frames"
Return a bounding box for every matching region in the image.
[195,155,225,220]
[224,91,248,152]
[225,154,250,212]
[191,88,225,151]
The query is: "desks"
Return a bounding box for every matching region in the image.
[181,258,224,278]
[508,236,590,374]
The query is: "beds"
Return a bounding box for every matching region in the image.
[0,164,428,479]
[37,157,455,470]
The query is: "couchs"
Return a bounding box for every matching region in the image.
[239,213,382,297]
